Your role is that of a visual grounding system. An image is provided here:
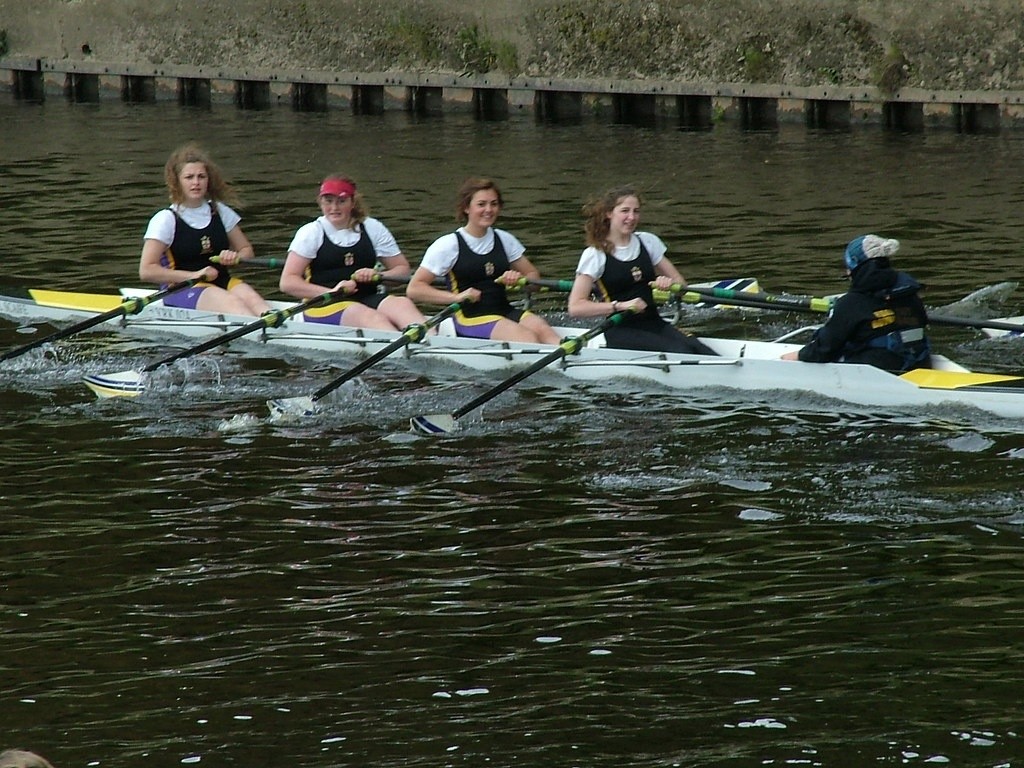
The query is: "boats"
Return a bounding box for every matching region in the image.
[1,277,1024,430]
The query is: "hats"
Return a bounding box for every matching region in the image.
[844,234,901,269]
[320,180,356,199]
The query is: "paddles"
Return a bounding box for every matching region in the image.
[83,286,346,398]
[493,275,827,314]
[209,255,285,269]
[651,281,1024,333]
[351,272,447,286]
[266,301,469,417]
[410,309,633,436]
[0,276,206,362]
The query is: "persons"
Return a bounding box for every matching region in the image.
[568,186,721,357]
[406,177,562,346]
[280,170,435,334]
[782,235,933,378]
[138,142,271,318]
